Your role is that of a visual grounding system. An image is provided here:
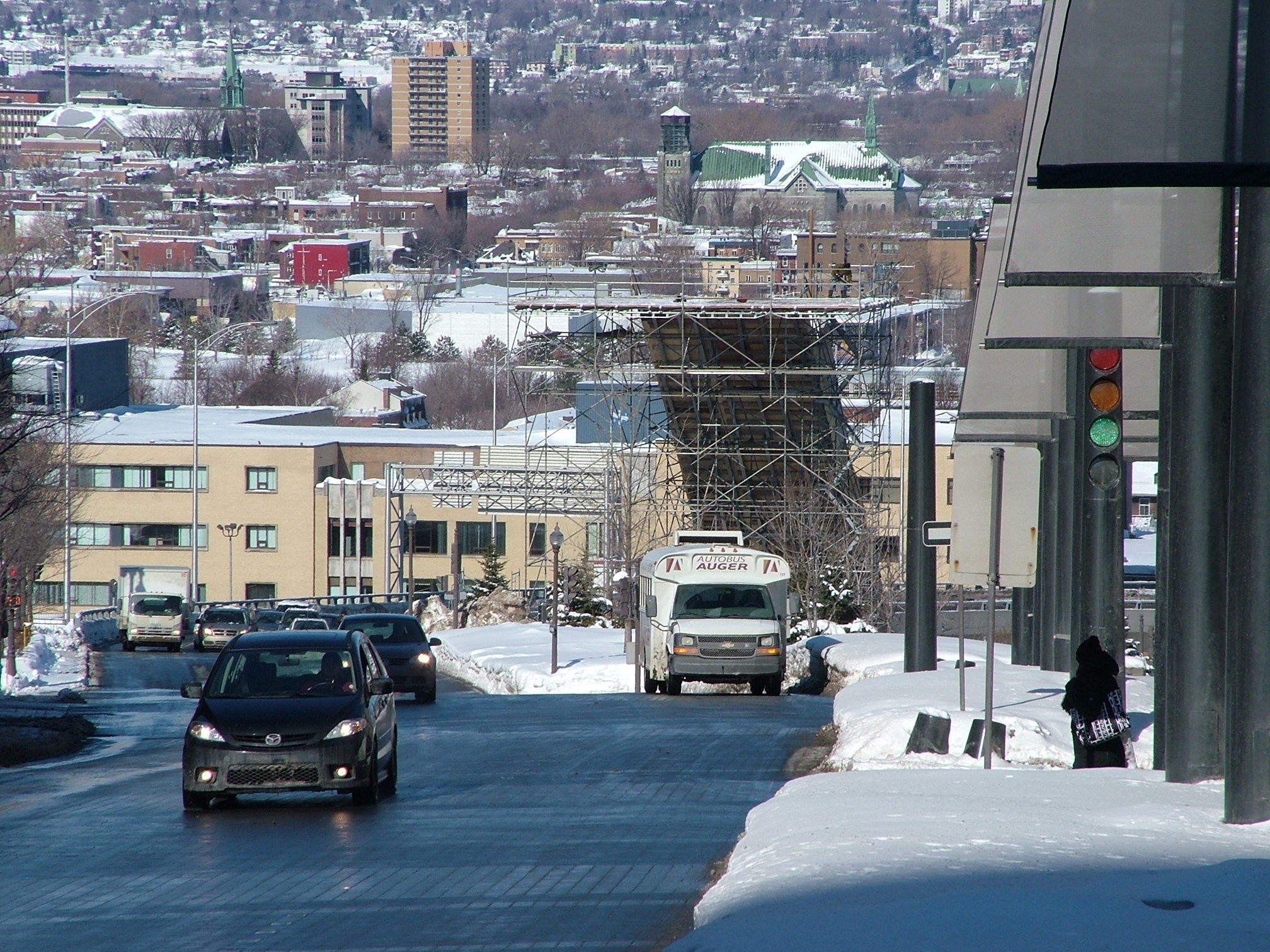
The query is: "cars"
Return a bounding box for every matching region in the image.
[178,632,400,813]
[335,613,443,704]
[190,601,393,653]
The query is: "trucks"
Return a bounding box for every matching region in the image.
[115,563,191,654]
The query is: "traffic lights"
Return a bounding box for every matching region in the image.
[7,566,19,588]
[7,594,21,608]
[32,563,44,582]
[1083,348,1123,501]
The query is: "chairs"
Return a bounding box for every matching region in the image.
[134,599,148,613]
[161,600,177,611]
[680,588,704,609]
[737,588,766,608]
[229,663,274,694]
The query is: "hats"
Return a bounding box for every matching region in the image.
[1075,634,1102,664]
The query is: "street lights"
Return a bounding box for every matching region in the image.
[399,256,416,269]
[64,286,174,626]
[192,318,279,615]
[217,523,243,602]
[445,247,463,298]
[404,503,418,615]
[549,520,565,673]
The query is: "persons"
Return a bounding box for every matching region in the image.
[336,610,347,626]
[1062,635,1128,767]
[297,651,353,693]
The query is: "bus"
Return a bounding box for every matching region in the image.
[634,525,794,697]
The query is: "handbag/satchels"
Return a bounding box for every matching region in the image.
[1069,672,1131,745]
